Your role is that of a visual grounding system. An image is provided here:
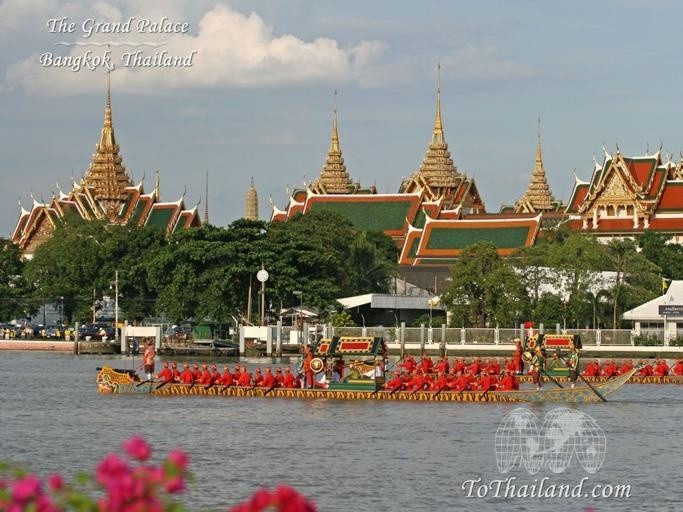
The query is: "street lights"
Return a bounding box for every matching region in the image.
[291,290,303,353]
[255,262,268,327]
[109,268,121,340]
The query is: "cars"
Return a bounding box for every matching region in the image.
[0,317,118,340]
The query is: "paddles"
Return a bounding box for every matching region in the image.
[561,356,608,402]
[519,350,563,388]
[372,375,501,398]
[136,375,281,395]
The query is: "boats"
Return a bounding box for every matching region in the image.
[93,366,637,405]
[348,356,683,386]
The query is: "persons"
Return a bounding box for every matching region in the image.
[0,318,185,342]
[142,340,683,390]
